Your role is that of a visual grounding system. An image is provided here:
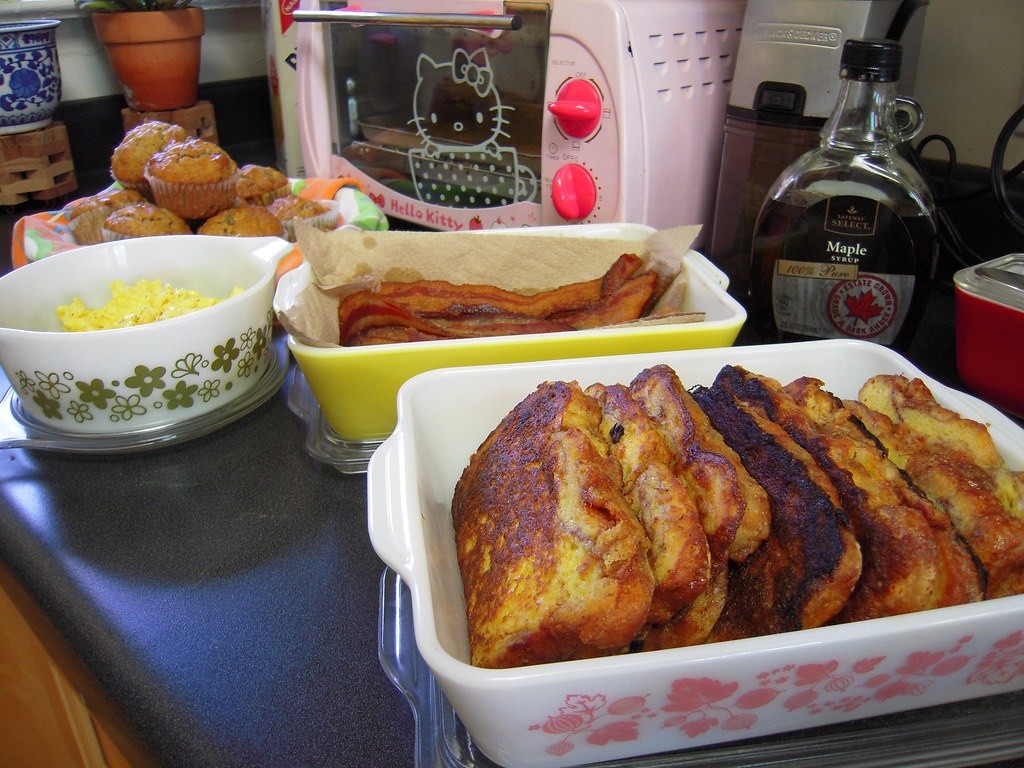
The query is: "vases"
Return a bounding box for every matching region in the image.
[0,20,63,136]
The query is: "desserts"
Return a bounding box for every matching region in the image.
[69,121,340,242]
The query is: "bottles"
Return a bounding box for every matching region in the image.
[746,38,945,363]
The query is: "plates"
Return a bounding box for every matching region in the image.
[9,182,391,270]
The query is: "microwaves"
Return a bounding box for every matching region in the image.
[290,1,746,255]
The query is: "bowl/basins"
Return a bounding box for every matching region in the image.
[366,335,1024,765]
[269,221,745,473]
[1,233,294,437]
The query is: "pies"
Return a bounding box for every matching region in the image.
[452,361,1024,670]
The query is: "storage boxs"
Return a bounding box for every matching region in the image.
[365,338,1024,768]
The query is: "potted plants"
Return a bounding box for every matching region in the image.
[74,0,206,111]
